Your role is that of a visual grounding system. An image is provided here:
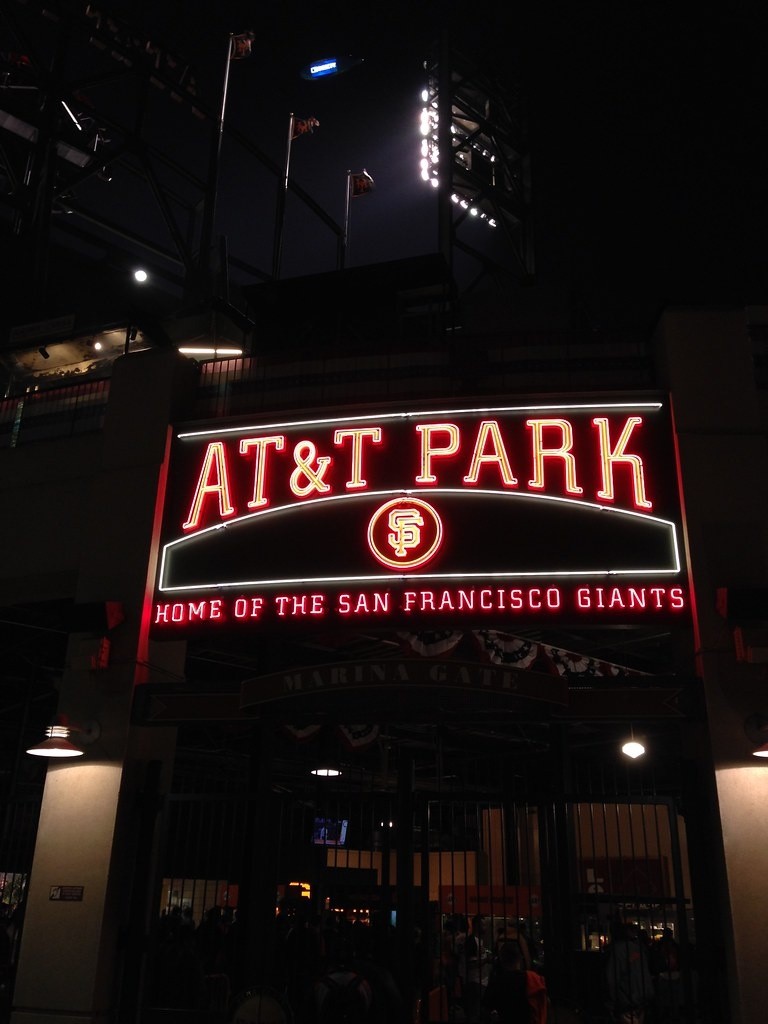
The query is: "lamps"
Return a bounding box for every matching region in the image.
[311,758,342,776]
[26,725,85,757]
[752,743,768,758]
[131,328,137,339]
[40,346,49,358]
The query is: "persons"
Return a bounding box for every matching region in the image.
[0,903,23,975]
[154,894,705,1024]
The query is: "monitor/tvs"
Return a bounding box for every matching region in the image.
[309,816,350,848]
